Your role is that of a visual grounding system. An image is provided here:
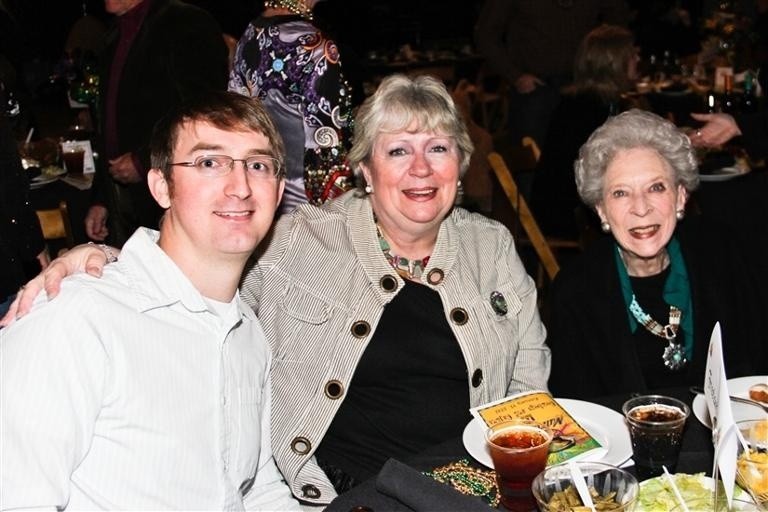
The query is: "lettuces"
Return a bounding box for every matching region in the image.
[637,473,740,512]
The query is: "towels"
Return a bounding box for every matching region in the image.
[375,456,499,512]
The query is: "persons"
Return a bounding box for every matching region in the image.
[0,72,550,510]
[542,106,766,396]
[2,2,765,295]
[1,89,297,511]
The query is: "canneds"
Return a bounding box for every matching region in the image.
[744,74,753,90]
[724,74,735,96]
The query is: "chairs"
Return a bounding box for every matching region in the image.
[487,151,577,289]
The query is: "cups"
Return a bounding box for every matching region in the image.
[621,393,692,477]
[485,420,554,512]
[62,146,86,182]
[720,417,767,506]
[529,460,641,512]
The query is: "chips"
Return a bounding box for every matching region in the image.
[545,485,623,512]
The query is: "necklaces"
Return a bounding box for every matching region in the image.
[617,247,690,368]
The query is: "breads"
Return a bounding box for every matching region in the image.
[736,416,767,503]
[749,383,768,404]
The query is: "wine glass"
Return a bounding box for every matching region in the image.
[17,139,36,165]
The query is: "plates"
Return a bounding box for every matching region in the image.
[459,397,636,479]
[619,472,758,511]
[689,373,767,450]
[687,163,751,183]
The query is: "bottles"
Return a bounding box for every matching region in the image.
[661,49,682,82]
[720,70,759,167]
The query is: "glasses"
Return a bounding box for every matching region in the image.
[165,153,283,181]
